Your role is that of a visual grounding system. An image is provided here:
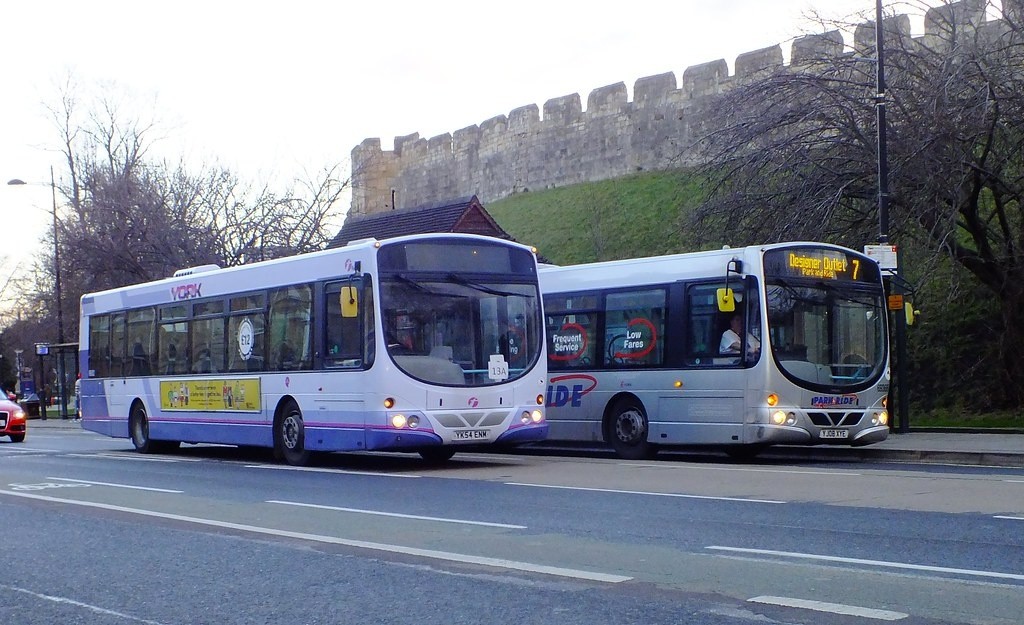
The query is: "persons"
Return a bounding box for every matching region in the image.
[718,314,760,356]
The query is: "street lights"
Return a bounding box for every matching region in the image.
[7,165,68,420]
[14,347,24,400]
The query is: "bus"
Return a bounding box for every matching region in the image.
[537,239,919,461]
[76,233,548,464]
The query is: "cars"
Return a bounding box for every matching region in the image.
[0,388,25,443]
